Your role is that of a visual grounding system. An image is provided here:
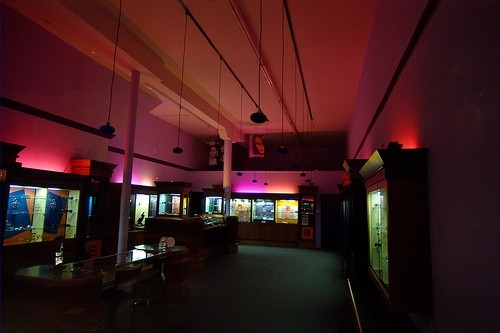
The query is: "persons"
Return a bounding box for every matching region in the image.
[253,135,264,156]
[210,145,217,164]
[219,144,224,161]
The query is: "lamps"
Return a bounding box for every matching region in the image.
[237,86,243,176]
[252,125,257,183]
[250,0,268,123]
[290,55,314,185]
[276,3,288,154]
[99,0,122,134]
[173,9,188,154]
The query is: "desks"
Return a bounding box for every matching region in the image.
[14,244,207,317]
[145,217,239,261]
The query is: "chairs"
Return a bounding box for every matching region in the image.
[102,267,175,333]
